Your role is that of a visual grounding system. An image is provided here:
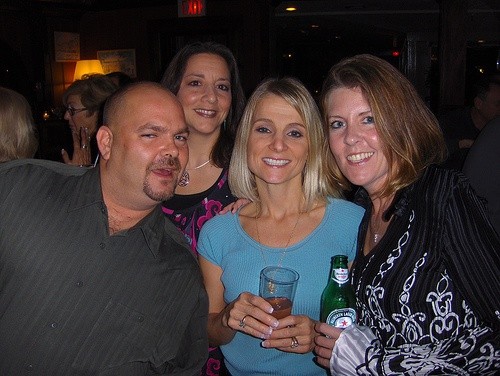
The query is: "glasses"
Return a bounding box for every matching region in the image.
[65,104,95,117]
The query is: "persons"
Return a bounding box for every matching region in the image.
[195,75,366,376]
[62,70,133,167]
[0,87,38,165]
[314,53,500,376]
[157,42,247,376]
[0,83,209,376]
[437,80,500,173]
[464,117,500,215]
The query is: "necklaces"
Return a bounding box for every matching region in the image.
[370,207,381,248]
[255,206,302,292]
[178,159,214,187]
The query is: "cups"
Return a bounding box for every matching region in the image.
[260,266,301,319]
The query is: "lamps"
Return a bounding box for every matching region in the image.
[73,59,105,81]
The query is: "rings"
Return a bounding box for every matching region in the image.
[239,315,248,328]
[82,144,87,148]
[291,337,298,349]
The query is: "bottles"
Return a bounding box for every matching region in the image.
[320,254,358,339]
